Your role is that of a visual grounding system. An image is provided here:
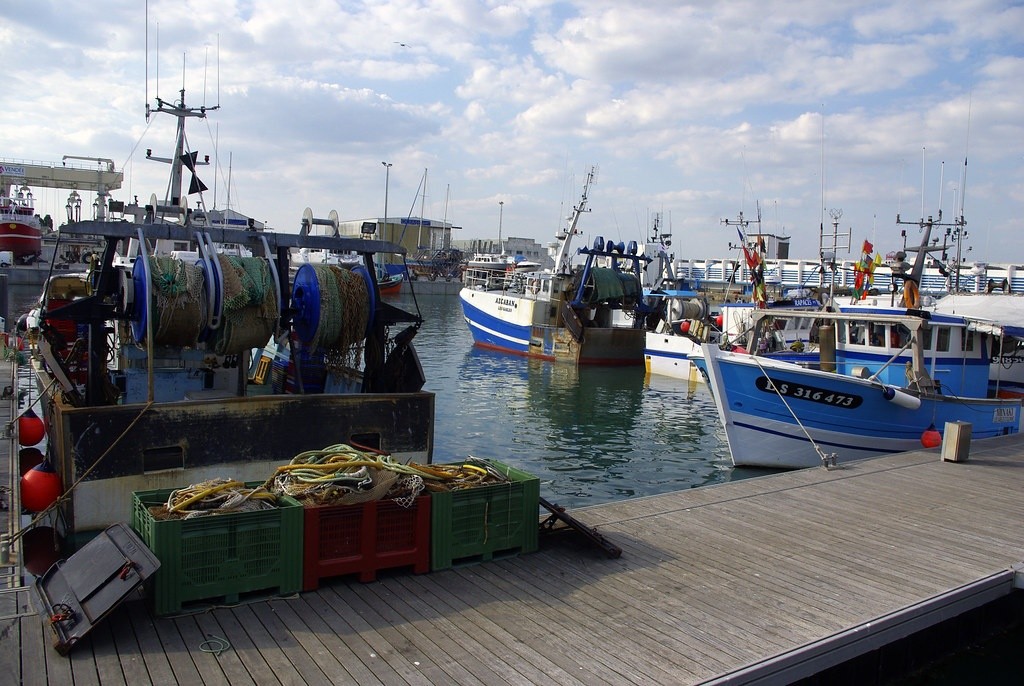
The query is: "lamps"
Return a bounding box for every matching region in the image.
[360,222,376,240]
[109,202,124,221]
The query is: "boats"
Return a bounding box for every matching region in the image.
[0,189,42,261]
[17,0,1024,546]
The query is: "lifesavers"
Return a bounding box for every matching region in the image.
[530,280,540,294]
[82,252,92,264]
[903,280,920,309]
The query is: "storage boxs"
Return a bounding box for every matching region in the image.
[132,481,304,616]
[424,459,540,572]
[304,492,432,592]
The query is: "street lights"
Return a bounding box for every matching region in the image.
[498,201,504,254]
[381,161,392,279]
